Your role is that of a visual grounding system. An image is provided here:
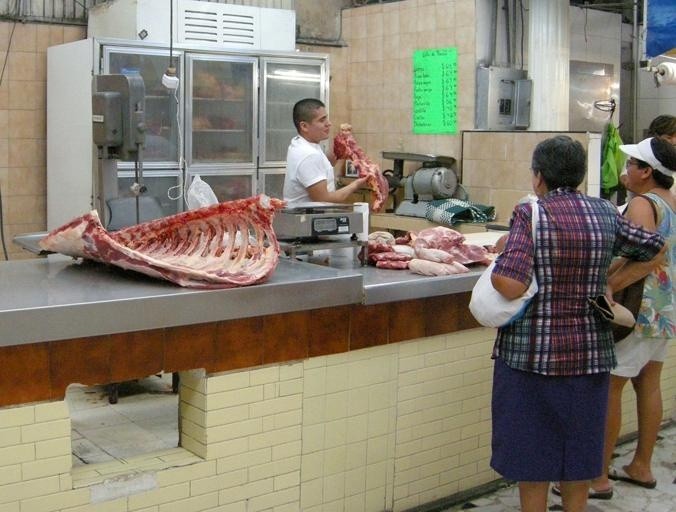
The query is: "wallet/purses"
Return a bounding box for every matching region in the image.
[590,290,637,329]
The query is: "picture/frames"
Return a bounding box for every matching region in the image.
[345,160,359,177]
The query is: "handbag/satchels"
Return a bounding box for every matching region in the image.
[465,242,542,329]
[611,269,644,343]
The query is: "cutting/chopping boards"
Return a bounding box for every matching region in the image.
[461,231,507,264]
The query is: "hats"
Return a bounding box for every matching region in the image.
[616,137,676,179]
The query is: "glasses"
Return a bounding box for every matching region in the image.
[626,157,646,168]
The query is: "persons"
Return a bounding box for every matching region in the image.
[551,137,676,498]
[282,99,370,210]
[619,114,676,203]
[489,135,668,510]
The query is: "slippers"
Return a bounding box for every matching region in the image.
[552,480,614,502]
[605,463,658,490]
